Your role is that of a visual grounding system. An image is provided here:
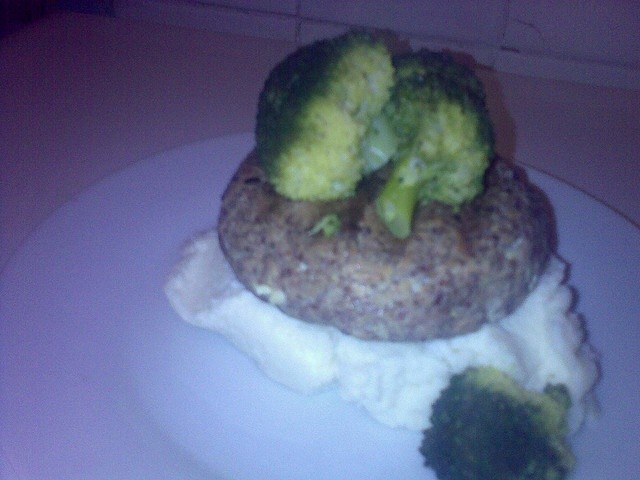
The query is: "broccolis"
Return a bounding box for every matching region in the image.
[372,48,497,239]
[419,365,576,477]
[256,27,398,203]
